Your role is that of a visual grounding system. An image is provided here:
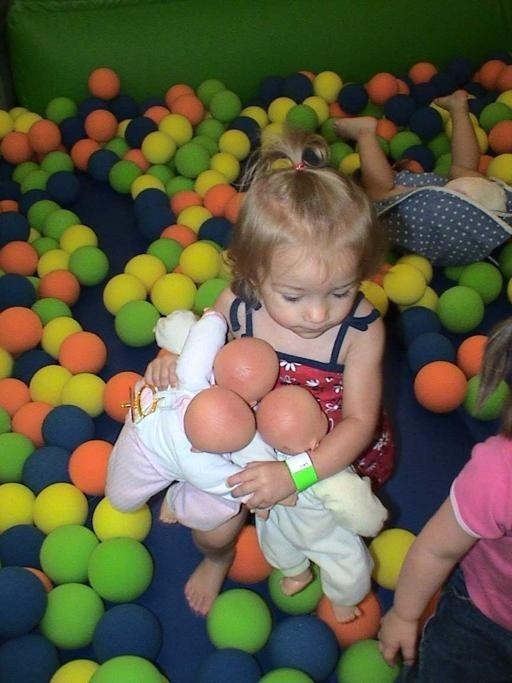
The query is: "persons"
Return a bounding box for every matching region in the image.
[105,307,388,623]
[379,318,512,683]
[333,90,512,267]
[146,129,396,615]
